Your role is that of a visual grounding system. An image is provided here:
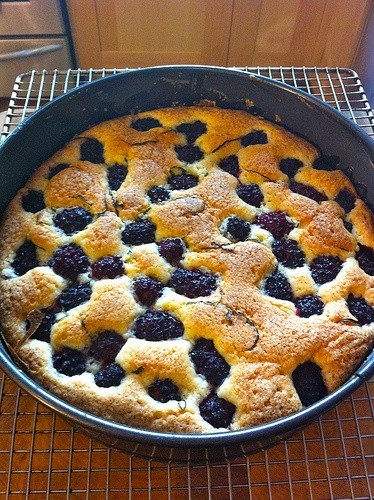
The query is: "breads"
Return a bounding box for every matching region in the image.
[0,107,374,436]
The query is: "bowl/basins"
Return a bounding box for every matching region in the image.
[0,64,373,462]
